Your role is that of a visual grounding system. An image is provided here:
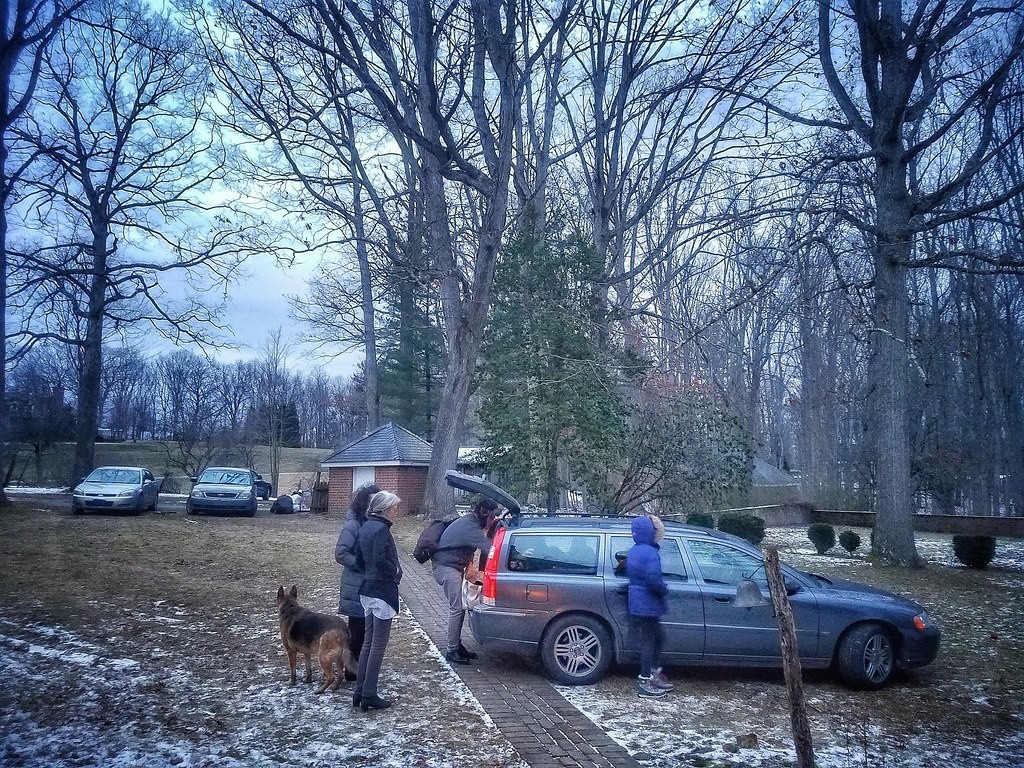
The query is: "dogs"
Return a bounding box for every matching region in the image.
[276,585,359,695]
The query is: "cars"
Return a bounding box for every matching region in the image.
[186,466,260,517]
[71,466,159,516]
[249,469,272,501]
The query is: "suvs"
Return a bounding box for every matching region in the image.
[443,468,941,688]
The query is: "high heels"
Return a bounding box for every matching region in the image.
[361,694,391,712]
[353,693,362,706]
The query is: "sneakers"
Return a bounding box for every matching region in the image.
[637,674,667,698]
[650,667,674,691]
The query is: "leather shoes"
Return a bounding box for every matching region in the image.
[458,651,478,659]
[447,650,470,664]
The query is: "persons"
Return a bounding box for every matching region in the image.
[431,499,535,665]
[352,491,402,711]
[335,485,381,682]
[625,515,675,699]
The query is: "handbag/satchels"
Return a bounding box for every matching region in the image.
[413,518,449,565]
